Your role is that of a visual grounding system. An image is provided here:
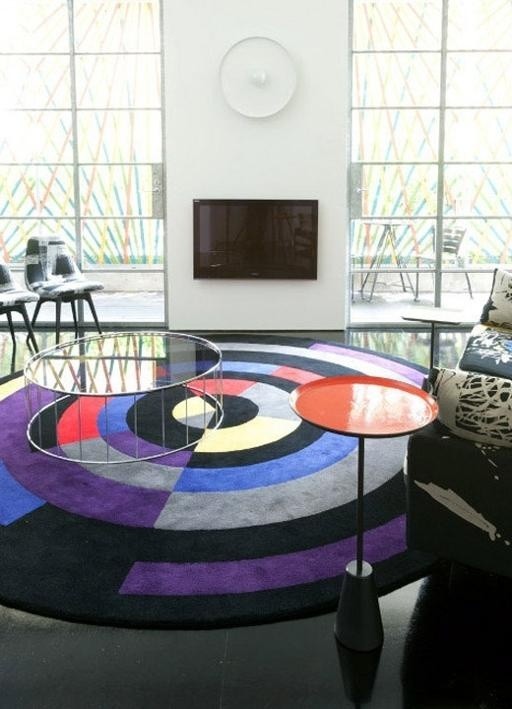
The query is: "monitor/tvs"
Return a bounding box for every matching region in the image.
[192,198,319,281]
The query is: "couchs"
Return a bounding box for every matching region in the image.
[401,267,512,580]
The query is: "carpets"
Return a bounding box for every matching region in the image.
[0,333,436,633]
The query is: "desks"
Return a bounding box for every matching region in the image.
[288,370,447,653]
[400,300,463,390]
[354,215,419,299]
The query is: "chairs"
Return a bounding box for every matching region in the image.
[2,236,103,377]
[414,226,477,298]
